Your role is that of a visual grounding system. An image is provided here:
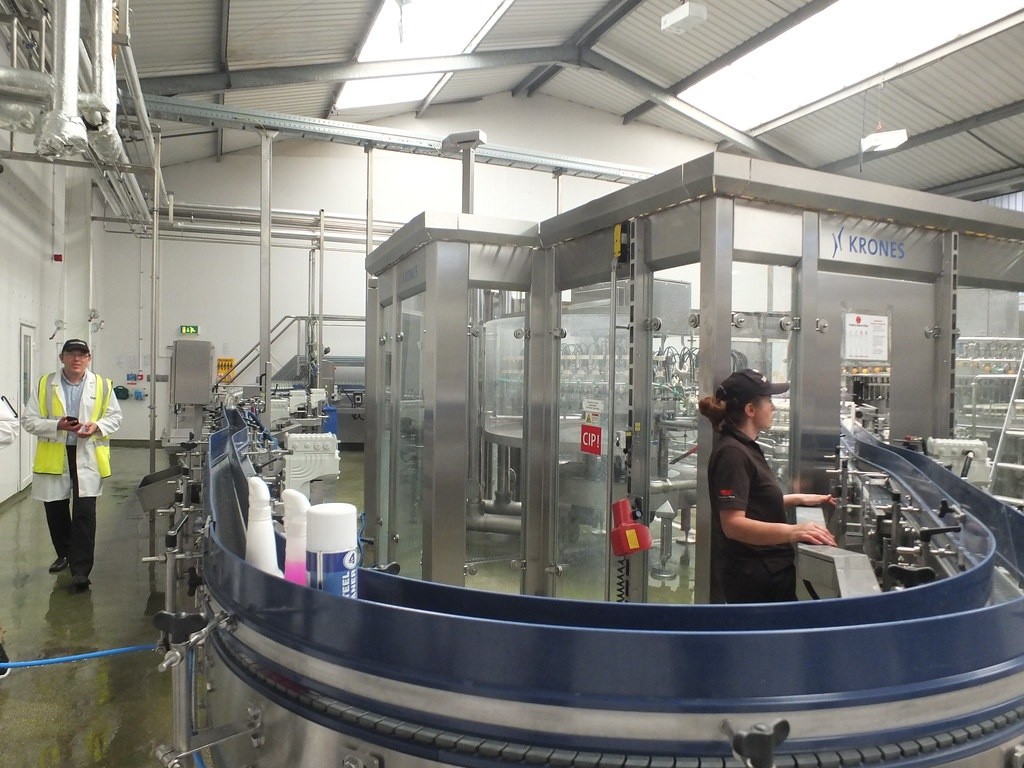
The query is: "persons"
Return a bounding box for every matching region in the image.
[1,397,20,681]
[21,338,125,589]
[701,369,839,605]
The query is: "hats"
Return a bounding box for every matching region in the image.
[719,368,791,408]
[62,339,90,353]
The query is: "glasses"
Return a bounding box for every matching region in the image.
[63,351,91,358]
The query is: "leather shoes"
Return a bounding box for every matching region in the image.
[49,556,69,571]
[72,575,92,585]
